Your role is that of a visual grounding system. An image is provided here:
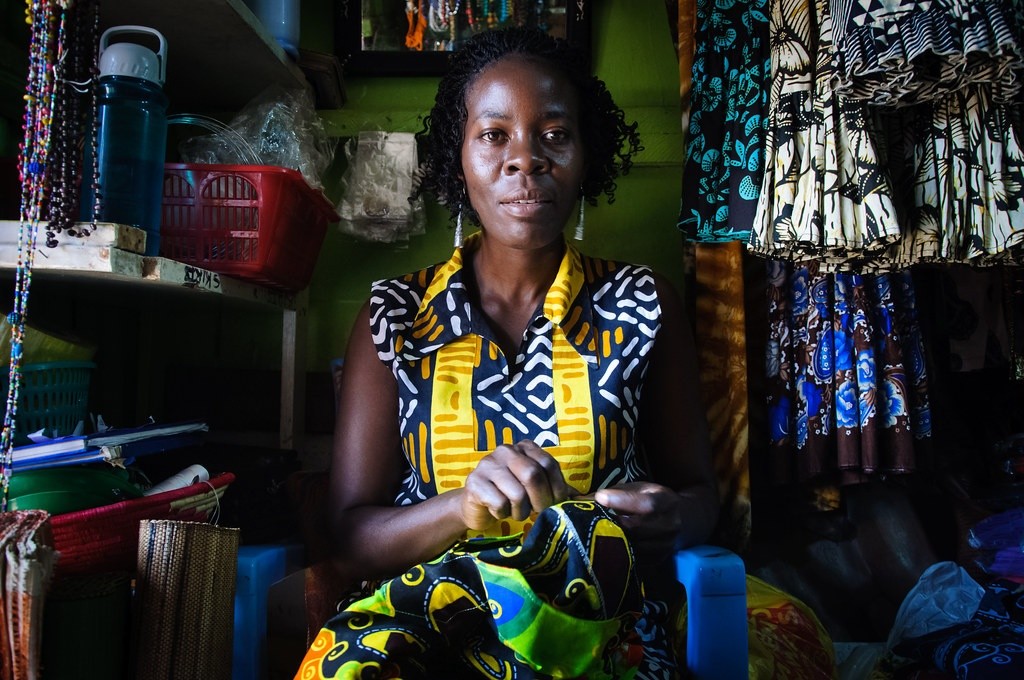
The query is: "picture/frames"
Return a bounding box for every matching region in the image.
[333,0,593,79]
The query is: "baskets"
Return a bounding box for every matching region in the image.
[0,360,96,440]
[46,474,235,569]
[161,163,342,292]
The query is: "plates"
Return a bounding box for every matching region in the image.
[0,468,146,515]
[166,112,264,262]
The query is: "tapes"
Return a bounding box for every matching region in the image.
[0,313,99,385]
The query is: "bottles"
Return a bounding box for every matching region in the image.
[78,25,168,257]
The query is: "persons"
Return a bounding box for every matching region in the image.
[289,26,722,680]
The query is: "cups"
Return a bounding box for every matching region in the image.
[245,0,301,64]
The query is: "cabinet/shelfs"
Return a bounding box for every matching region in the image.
[0,0,319,459]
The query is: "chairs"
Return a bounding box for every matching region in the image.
[224,355,750,680]
